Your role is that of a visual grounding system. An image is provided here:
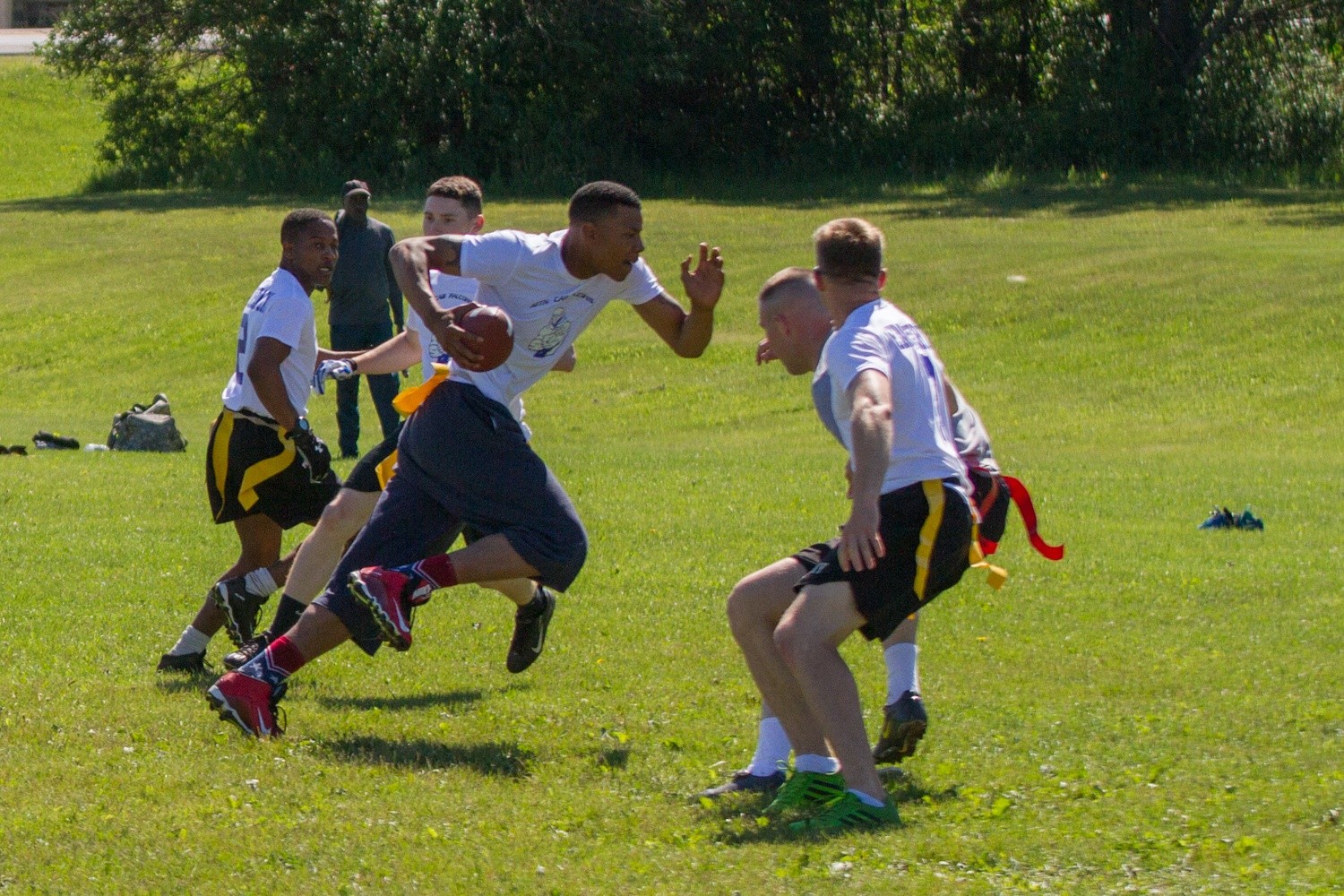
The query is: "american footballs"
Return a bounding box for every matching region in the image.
[453,306,514,373]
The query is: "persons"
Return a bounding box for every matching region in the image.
[157,177,576,674]
[705,266,1010,795]
[726,216,981,838]
[206,179,725,738]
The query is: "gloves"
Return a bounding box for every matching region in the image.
[293,429,332,484]
[312,358,358,395]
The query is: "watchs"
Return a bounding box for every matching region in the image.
[284,417,310,439]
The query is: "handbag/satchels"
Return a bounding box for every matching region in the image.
[107,393,188,453]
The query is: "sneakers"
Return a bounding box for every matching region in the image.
[506,584,556,673]
[206,671,287,740]
[212,575,270,649]
[223,630,276,669]
[789,792,903,839]
[688,769,787,806]
[156,648,220,681]
[761,759,850,823]
[346,565,432,652]
[872,689,928,764]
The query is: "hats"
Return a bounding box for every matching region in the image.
[342,180,371,198]
